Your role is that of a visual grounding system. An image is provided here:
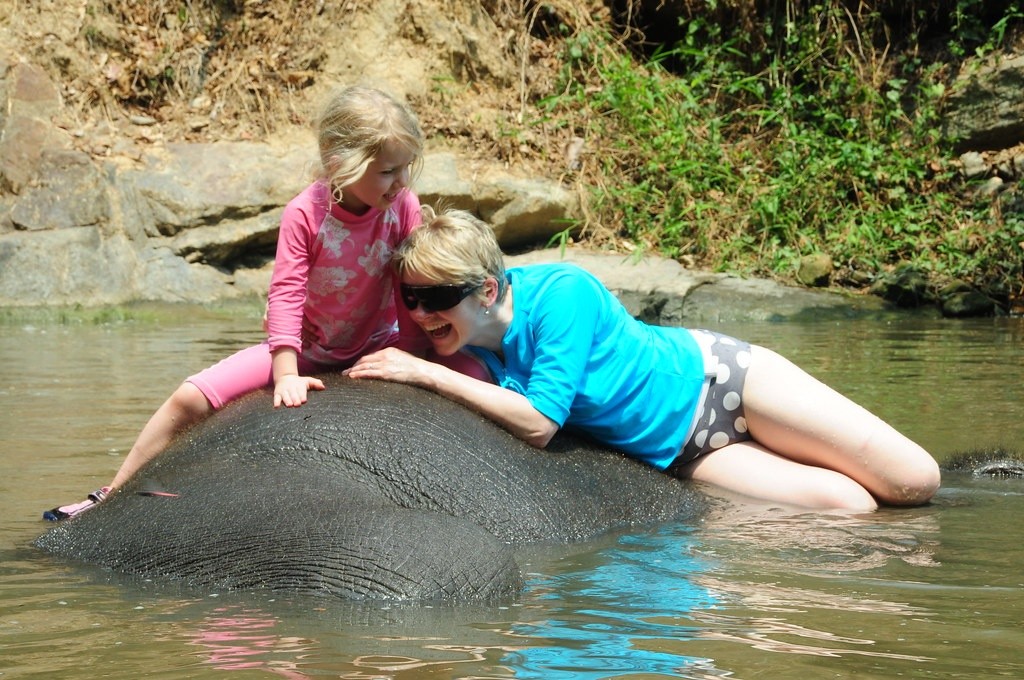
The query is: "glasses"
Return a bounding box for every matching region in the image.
[400,283,483,311]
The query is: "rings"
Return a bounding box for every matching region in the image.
[369,362,373,369]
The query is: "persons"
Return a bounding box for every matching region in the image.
[42,86,495,523]
[342,209,941,513]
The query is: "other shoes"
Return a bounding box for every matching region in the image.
[43,487,109,522]
